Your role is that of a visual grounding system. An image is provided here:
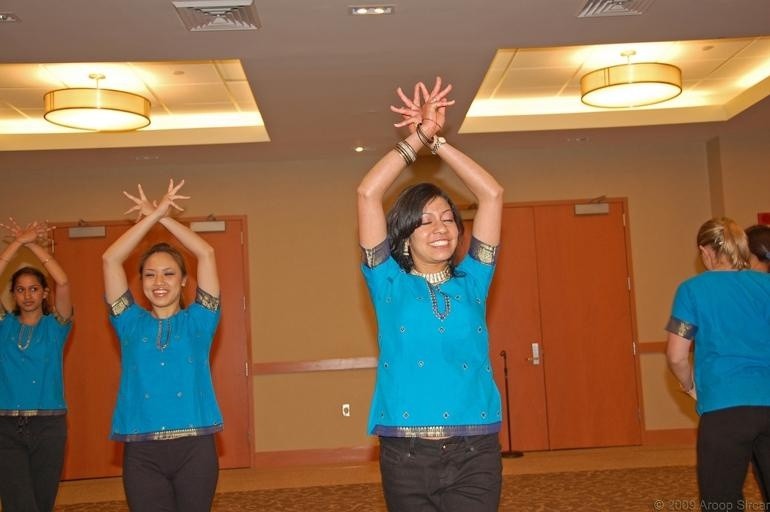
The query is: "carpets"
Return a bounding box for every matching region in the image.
[54,460,767,512]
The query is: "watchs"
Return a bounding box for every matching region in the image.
[430,136,446,155]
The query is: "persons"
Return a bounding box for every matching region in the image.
[665,218,769,512]
[356,74,505,512]
[744,223,770,269]
[101,177,221,512]
[0,216,75,512]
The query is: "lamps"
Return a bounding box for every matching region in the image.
[575,49,683,112]
[40,71,153,134]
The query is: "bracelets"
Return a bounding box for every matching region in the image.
[390,138,418,166]
[680,380,693,393]
[41,255,57,265]
[1,257,9,263]
[414,121,436,146]
